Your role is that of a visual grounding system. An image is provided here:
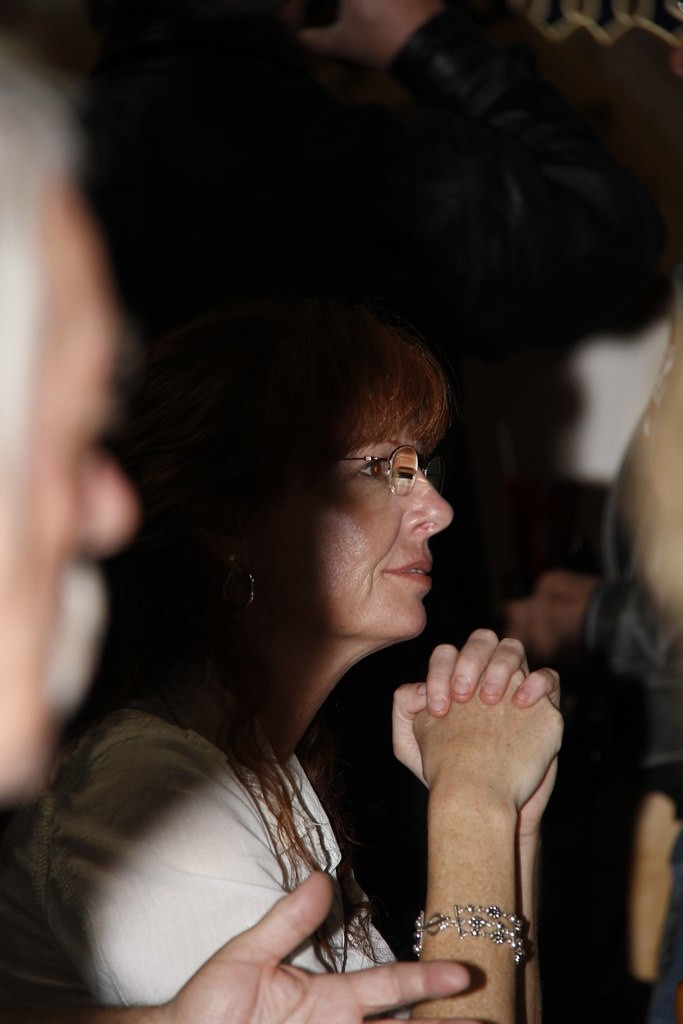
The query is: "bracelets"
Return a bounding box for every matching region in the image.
[410,904,531,966]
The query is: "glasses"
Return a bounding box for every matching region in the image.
[314,444,446,504]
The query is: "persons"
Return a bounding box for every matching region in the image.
[83,0,669,626]
[503,298,683,1024]
[0,300,566,1024]
[0,48,482,1024]
